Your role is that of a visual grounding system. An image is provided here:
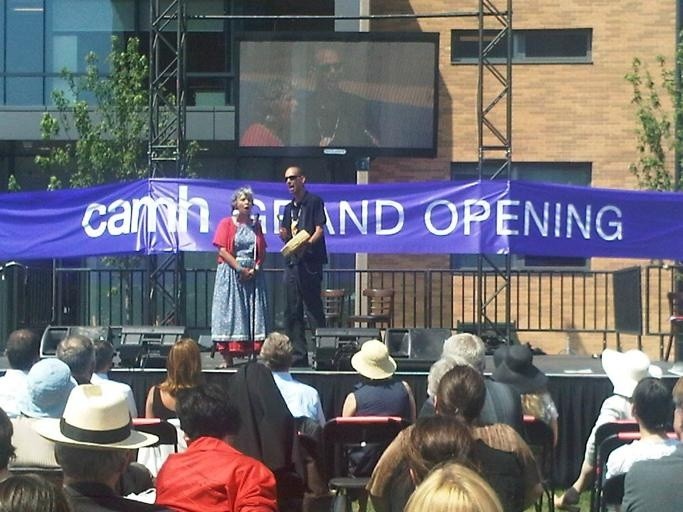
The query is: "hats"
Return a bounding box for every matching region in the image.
[18,357,78,419]
[35,384,159,450]
[350,338,397,381]
[601,347,663,399]
[490,345,549,392]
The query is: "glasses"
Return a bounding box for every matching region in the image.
[318,61,340,72]
[284,176,301,183]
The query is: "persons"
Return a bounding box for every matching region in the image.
[278,166,327,366]
[1,328,682,512]
[304,46,383,148]
[212,185,268,369]
[240,78,299,147]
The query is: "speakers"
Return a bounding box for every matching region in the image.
[384,328,453,372]
[118,326,183,368]
[37,325,112,364]
[314,327,381,373]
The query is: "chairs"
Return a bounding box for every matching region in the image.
[322,413,413,512]
[346,285,395,329]
[129,417,178,455]
[302,288,347,333]
[590,418,679,512]
[520,414,556,512]
[664,292,683,362]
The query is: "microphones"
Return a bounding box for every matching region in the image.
[252,213,260,227]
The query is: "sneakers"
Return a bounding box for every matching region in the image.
[216,358,233,368]
